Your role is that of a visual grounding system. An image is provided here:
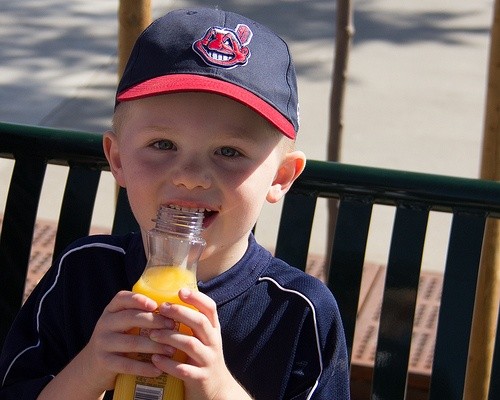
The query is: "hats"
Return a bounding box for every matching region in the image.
[114,8,299,140]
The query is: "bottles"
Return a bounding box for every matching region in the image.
[112,206,207,400]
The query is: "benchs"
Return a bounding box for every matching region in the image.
[0,119,500,400]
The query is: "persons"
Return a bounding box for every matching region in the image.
[2,8,351,400]
